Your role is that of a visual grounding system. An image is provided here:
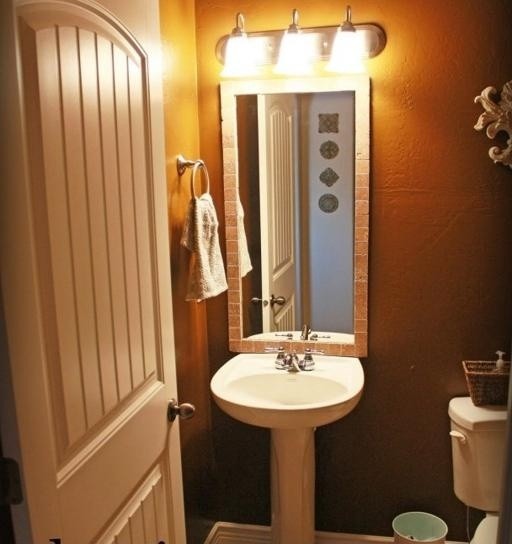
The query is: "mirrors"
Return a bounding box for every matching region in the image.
[216,73,371,358]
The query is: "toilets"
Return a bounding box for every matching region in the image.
[448,396,508,544]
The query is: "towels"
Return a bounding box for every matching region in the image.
[237,197,253,280]
[178,193,229,304]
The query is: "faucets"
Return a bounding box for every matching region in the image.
[301,322,311,340]
[282,348,301,372]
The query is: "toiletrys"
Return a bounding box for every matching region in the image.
[491,350,508,374]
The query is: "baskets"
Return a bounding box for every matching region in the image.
[462,361,509,406]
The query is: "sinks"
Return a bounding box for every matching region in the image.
[246,331,355,344]
[209,353,365,429]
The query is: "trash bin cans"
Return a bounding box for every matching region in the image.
[392,512,448,544]
